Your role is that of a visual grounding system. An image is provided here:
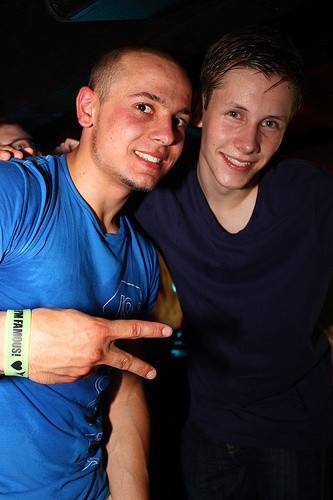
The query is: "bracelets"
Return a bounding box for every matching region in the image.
[4,307,30,379]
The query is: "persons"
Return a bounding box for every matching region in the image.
[0,31,332,499]
[0,47,192,500]
[0,122,34,161]
